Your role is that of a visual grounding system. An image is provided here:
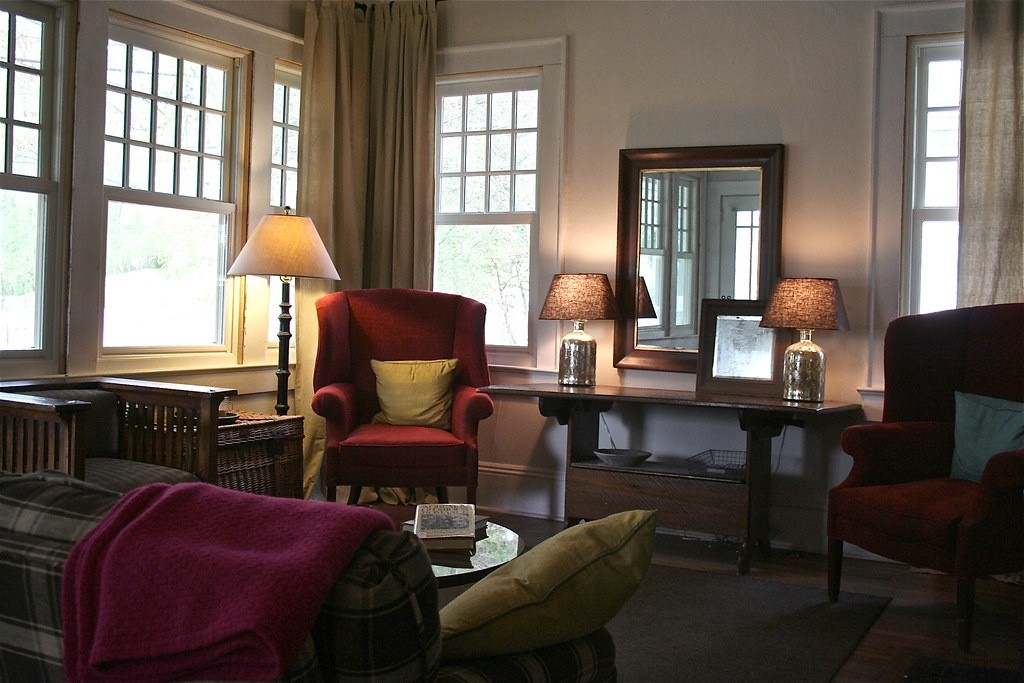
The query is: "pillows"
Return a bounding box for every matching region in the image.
[949,393,1024,482]
[371,357,457,432]
[437,510,658,659]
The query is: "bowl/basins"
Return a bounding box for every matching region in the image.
[592,449,653,466]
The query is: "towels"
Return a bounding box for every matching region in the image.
[60,481,397,683]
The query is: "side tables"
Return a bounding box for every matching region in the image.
[123,411,305,496]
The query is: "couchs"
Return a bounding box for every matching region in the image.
[0,470,618,683]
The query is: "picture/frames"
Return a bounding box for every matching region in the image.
[696,297,798,401]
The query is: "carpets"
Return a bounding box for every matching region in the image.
[523,548,892,683]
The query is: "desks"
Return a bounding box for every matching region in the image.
[475,382,860,575]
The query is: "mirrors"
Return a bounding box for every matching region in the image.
[614,144,783,373]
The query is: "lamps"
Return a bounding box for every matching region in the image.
[638,277,657,320]
[538,273,616,384]
[227,209,341,414]
[758,280,852,402]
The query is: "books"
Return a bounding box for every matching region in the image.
[401,503,490,552]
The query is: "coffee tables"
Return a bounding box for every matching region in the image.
[358,502,566,588]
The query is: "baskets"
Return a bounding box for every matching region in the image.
[687,448,749,481]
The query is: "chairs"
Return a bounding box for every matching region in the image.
[312,289,493,503]
[827,304,1024,650]
[0,371,239,495]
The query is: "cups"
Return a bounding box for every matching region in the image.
[218,396,234,416]
